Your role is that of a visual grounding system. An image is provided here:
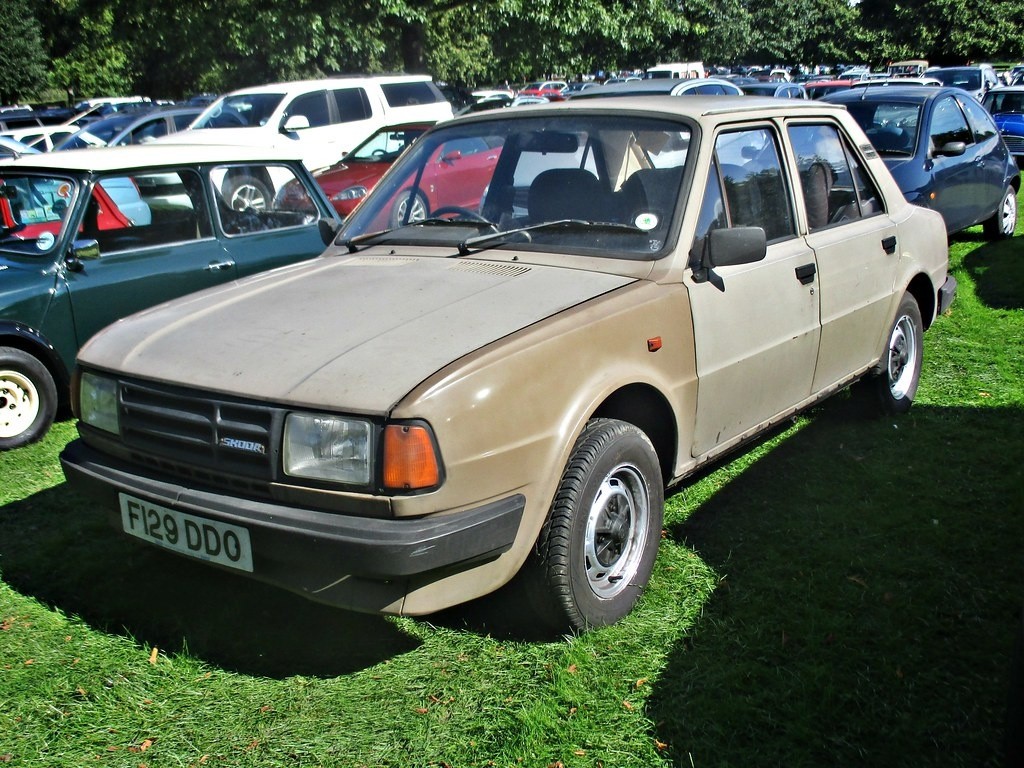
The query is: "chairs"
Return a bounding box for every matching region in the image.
[711,163,766,230]
[620,167,679,226]
[525,167,600,228]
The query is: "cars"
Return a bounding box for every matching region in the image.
[2,55,1024,254]
[59,95,954,642]
[0,146,348,452]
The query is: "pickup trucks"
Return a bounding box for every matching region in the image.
[139,72,454,215]
